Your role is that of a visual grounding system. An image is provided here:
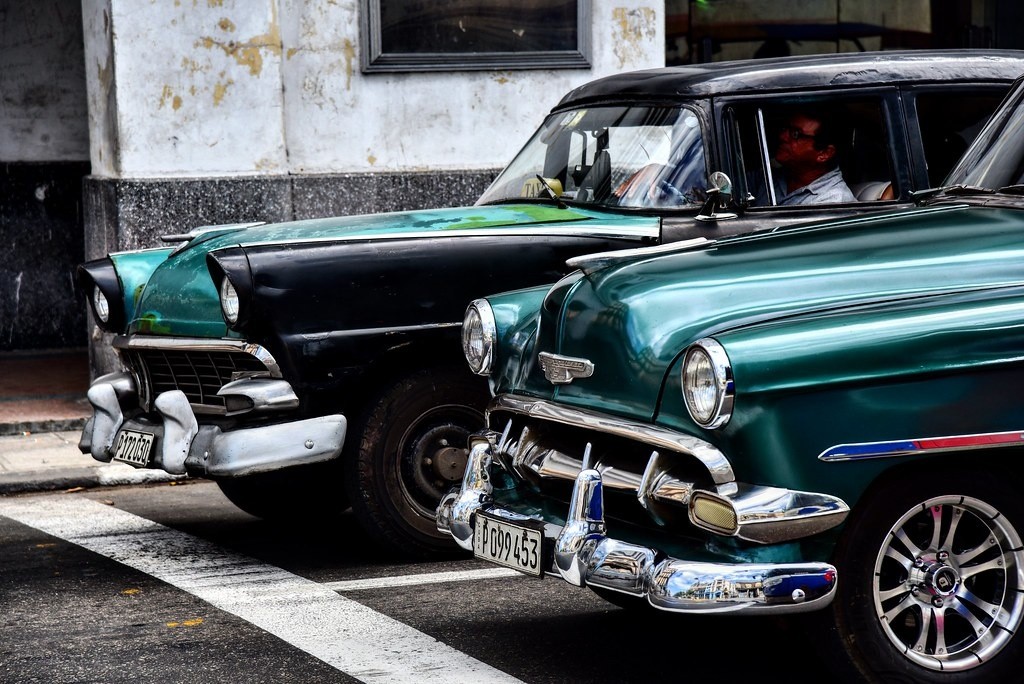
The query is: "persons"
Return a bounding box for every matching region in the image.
[748,107,858,202]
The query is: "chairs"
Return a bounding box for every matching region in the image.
[854,179,896,203]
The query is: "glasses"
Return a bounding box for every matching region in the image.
[782,124,820,140]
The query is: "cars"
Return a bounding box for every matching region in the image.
[432,67,1023,684]
[71,45,1023,561]
